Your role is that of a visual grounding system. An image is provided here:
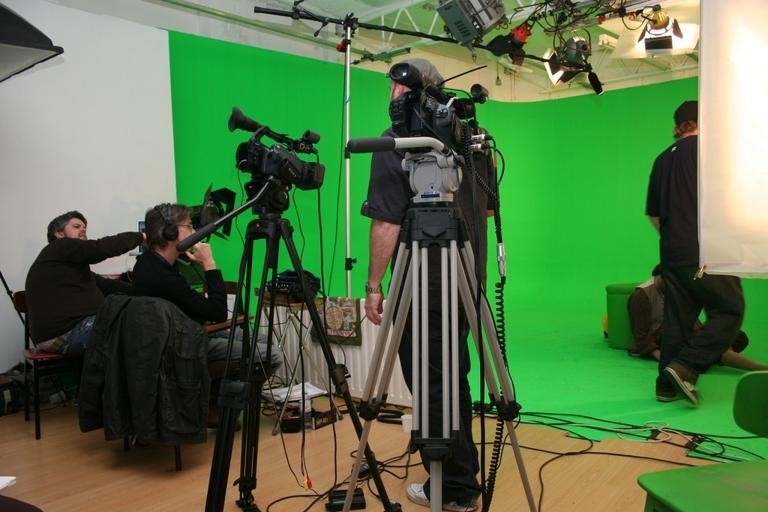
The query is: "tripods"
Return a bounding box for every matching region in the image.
[206,224,404,512]
[346,212,538,512]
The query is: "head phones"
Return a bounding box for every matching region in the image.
[154,203,178,241]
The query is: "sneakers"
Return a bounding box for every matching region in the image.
[655,379,684,401]
[407,482,479,512]
[663,360,699,404]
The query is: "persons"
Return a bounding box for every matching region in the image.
[358,56,500,511]
[131,203,283,431]
[24,209,149,356]
[626,263,768,373]
[645,100,745,405]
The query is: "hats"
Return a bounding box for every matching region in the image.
[387,57,443,88]
[673,100,696,123]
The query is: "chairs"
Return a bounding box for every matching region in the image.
[12,291,213,470]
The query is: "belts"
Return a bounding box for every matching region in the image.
[49,331,69,353]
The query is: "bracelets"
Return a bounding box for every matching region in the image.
[365,282,382,294]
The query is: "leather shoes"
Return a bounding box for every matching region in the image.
[207,408,241,431]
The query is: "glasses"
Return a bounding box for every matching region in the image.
[181,223,194,230]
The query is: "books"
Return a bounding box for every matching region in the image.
[262,380,328,402]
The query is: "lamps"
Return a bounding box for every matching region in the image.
[542,35,591,89]
[635,7,685,53]
